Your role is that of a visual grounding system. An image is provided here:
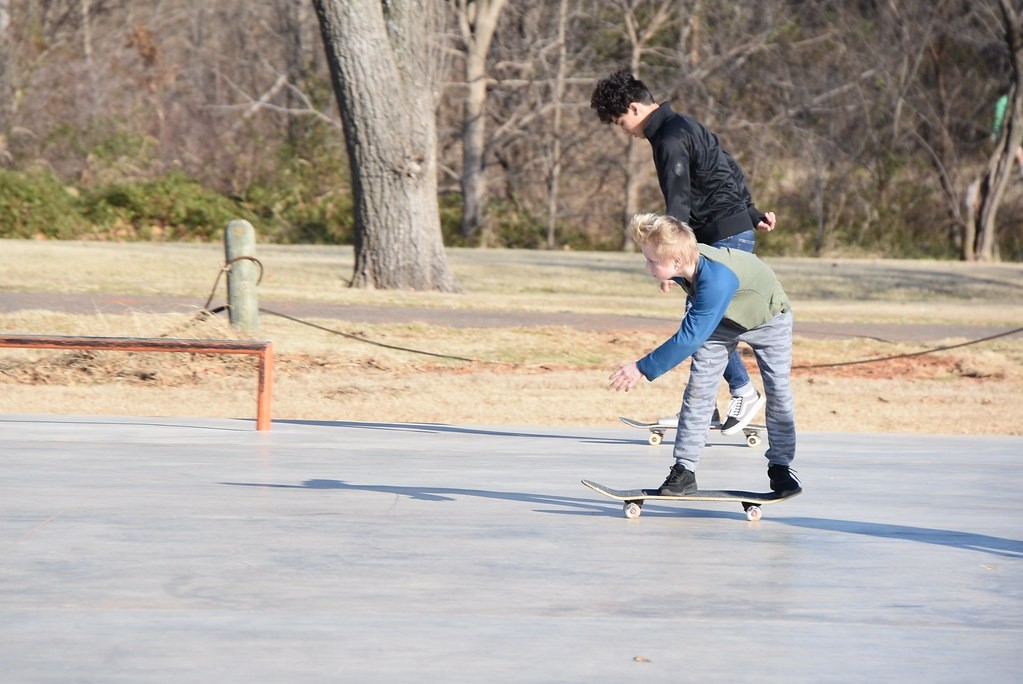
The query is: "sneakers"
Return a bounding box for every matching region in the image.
[723,389,767,435]
[767,465,801,497]
[660,464,697,497]
[657,408,722,427]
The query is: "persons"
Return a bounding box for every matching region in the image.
[590,71,777,436]
[608,212,802,498]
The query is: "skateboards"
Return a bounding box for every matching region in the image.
[579,478,804,521]
[618,415,769,447]
